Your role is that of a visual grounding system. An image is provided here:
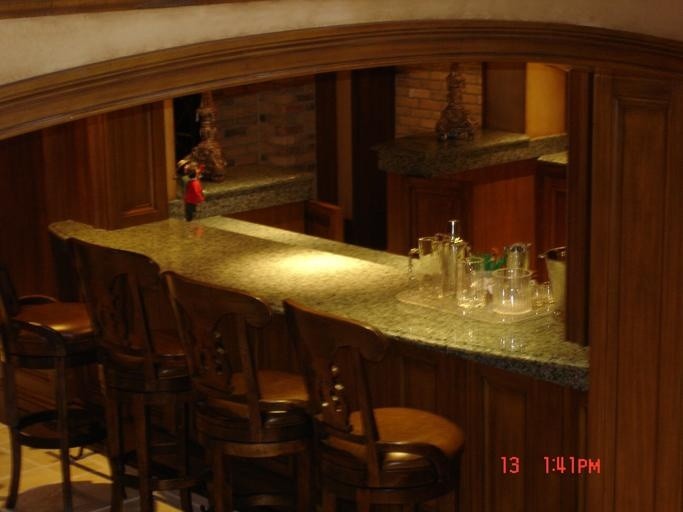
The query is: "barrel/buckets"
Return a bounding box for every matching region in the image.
[538,246,567,312]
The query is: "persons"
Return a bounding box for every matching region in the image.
[177,159,203,224]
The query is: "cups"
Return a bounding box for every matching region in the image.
[407,236,566,316]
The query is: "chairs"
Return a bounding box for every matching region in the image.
[1,237,127,511]
[283,299,467,510]
[161,269,326,510]
[65,235,208,511]
